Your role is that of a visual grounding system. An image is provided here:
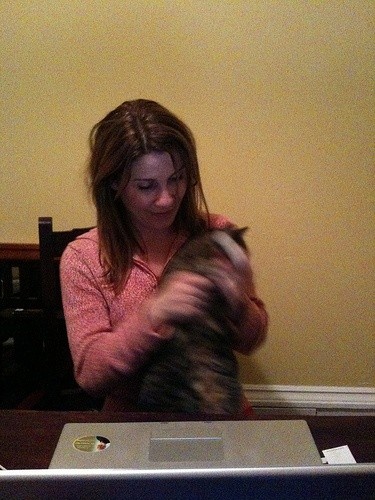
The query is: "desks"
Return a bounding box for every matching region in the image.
[0,411,375,470]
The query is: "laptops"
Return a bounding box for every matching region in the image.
[46,421,323,470]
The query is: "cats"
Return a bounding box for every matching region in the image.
[102,224,250,413]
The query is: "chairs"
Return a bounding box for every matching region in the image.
[12,217,109,411]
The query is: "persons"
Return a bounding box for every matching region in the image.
[61,98,270,420]
